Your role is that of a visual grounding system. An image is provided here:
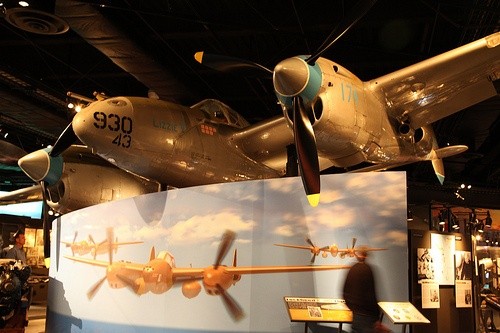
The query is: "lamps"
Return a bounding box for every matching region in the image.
[437,211,492,231]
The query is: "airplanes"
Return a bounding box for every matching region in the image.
[1,19,500,273]
[61,228,390,324]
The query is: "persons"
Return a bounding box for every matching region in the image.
[342,245,381,333]
[5,228,28,321]
